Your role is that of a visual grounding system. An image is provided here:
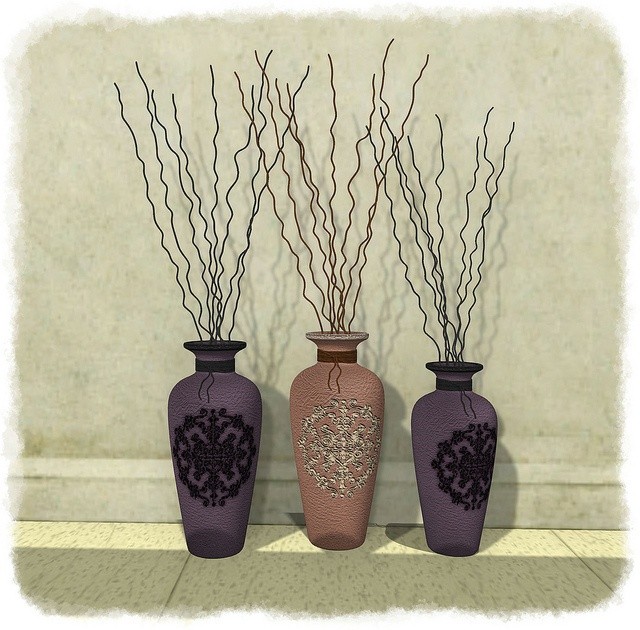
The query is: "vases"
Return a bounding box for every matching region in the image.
[168,341,261,559]
[288,332,385,550]
[411,363,499,556]
